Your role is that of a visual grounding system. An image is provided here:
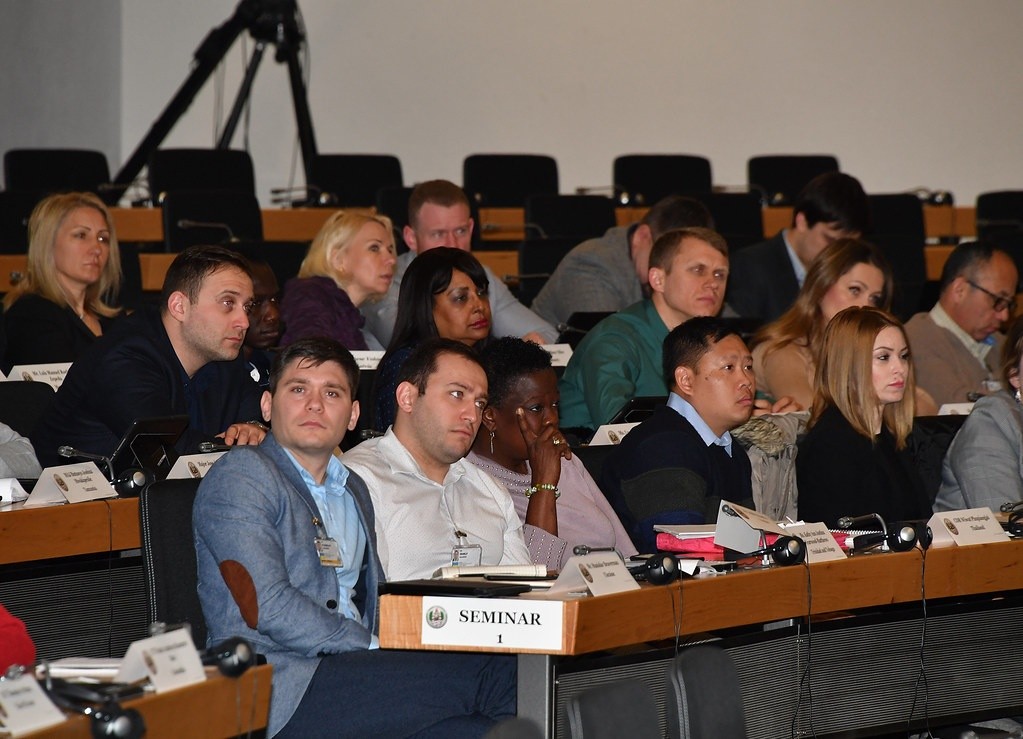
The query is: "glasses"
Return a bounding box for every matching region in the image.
[965,280,1017,313]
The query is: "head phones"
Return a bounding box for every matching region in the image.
[131,192,166,207]
[616,192,644,208]
[198,636,253,679]
[291,192,339,207]
[922,192,953,206]
[628,552,678,587]
[724,534,805,566]
[110,467,148,499]
[853,521,933,553]
[43,685,147,739]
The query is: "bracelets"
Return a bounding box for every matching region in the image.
[247,420,270,432]
[525,483,561,500]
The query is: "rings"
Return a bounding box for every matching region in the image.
[553,435,560,444]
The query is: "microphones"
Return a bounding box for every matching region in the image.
[199,443,235,453]
[837,513,888,548]
[483,222,547,239]
[10,272,21,278]
[360,429,384,440]
[150,621,184,634]
[572,545,626,563]
[504,273,552,281]
[271,186,323,195]
[99,183,158,207]
[58,445,115,491]
[558,325,588,335]
[577,184,625,195]
[967,393,985,402]
[177,219,237,243]
[7,660,52,690]
[1000,501,1023,513]
[722,504,770,566]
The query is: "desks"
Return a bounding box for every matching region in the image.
[0,204,1023,739]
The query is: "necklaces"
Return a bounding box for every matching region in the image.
[80,311,89,319]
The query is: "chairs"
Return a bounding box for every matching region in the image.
[662,642,748,739]
[519,195,618,308]
[464,154,560,207]
[308,154,405,208]
[976,190,1023,294]
[0,190,33,255]
[746,154,842,208]
[904,414,969,505]
[148,150,260,207]
[161,187,262,253]
[377,185,481,256]
[565,677,661,739]
[704,195,765,252]
[138,477,208,650]
[482,719,547,739]
[570,446,618,486]
[100,243,143,311]
[865,195,927,283]
[613,155,713,205]
[5,147,113,205]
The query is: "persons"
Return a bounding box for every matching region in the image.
[275,180,560,434]
[452,550,459,561]
[316,541,323,551]
[609,307,931,555]
[22,372,34,381]
[902,239,1023,516]
[0,420,43,479]
[0,603,36,678]
[189,337,518,739]
[559,225,893,432]
[186,240,281,400]
[0,192,132,365]
[30,246,271,479]
[336,337,533,582]
[528,172,907,334]
[471,337,719,657]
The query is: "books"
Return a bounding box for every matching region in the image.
[432,564,556,588]
[0,673,67,738]
[36,657,122,679]
[829,530,883,550]
[653,520,806,562]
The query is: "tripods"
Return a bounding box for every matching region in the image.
[106,0,317,207]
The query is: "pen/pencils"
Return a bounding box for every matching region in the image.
[454,574,484,578]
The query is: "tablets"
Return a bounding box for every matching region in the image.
[607,396,670,425]
[101,414,188,482]
[554,311,615,351]
